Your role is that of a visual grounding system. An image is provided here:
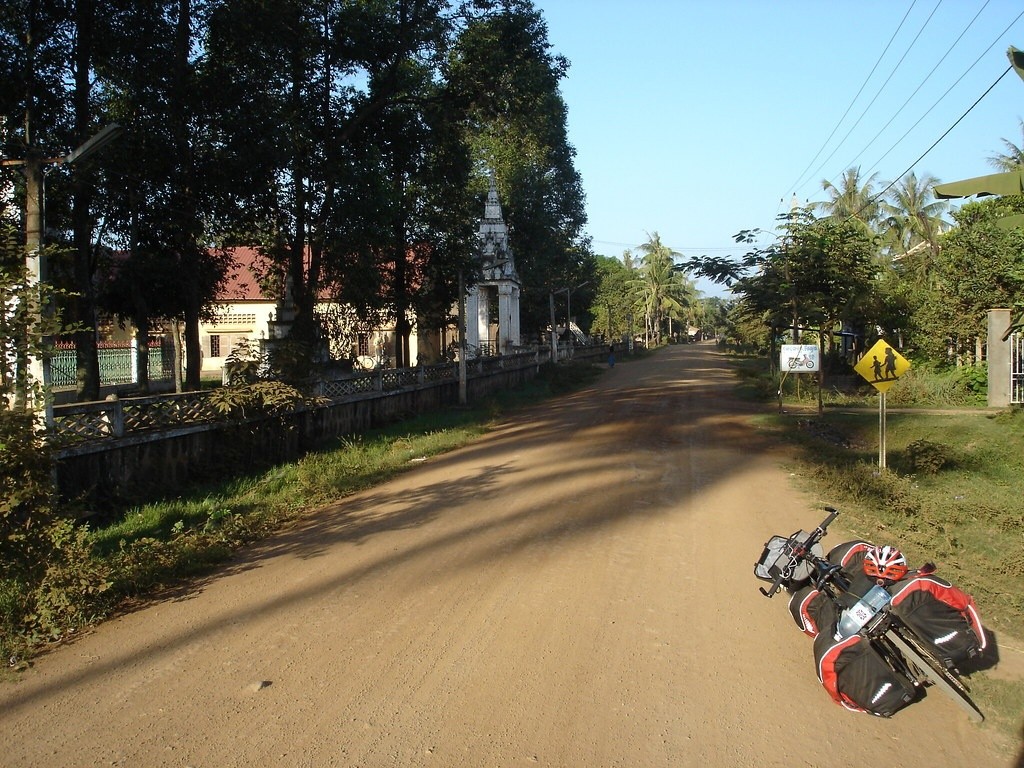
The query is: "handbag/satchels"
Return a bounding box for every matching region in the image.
[813,620,918,718]
[789,584,840,638]
[825,540,876,584]
[753,530,824,589]
[886,560,987,668]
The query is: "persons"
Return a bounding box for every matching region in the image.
[101,394,118,437]
[609,346,615,367]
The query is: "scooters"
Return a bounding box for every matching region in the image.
[788,353,814,369]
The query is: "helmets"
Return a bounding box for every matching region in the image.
[864,545,908,585]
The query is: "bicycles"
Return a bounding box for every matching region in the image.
[758,506,986,725]
